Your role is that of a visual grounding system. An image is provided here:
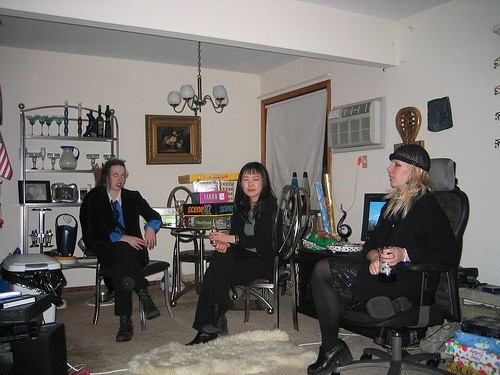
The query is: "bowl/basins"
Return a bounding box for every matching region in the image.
[97,292,110,301]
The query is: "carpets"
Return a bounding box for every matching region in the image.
[127,329,318,375]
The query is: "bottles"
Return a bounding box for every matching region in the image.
[96,105,112,138]
[210,224,219,245]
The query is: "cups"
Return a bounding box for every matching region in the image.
[377,246,395,274]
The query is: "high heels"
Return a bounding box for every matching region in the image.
[307,340,353,375]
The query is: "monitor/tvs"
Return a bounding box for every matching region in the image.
[361,193,390,240]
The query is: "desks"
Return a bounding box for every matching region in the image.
[159,222,228,307]
[145,114,202,165]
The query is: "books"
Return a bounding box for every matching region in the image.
[0,290,37,310]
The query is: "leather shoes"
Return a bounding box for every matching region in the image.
[185,330,217,345]
[203,304,225,334]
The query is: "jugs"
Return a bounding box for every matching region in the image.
[58,146,80,170]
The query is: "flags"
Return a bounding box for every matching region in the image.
[0,132,13,180]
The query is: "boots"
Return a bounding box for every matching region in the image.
[117,314,133,342]
[138,288,160,320]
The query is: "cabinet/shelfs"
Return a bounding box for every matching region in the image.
[296,248,461,344]
[19,103,119,270]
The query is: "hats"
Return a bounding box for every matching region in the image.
[389,145,431,173]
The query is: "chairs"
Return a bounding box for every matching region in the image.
[331,158,470,375]
[78,185,311,332]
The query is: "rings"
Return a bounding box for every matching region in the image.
[393,254,395,258]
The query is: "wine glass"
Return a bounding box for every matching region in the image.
[26,114,64,136]
[28,148,115,170]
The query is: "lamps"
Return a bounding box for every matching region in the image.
[168,41,229,117]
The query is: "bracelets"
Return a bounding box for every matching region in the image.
[402,248,407,262]
[235,235,239,245]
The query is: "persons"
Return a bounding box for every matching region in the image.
[80,158,162,342]
[185,162,283,346]
[307,144,457,375]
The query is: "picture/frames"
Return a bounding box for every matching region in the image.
[51,183,79,203]
[18,180,52,204]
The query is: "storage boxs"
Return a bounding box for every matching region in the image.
[144,172,240,229]
[445,315,500,375]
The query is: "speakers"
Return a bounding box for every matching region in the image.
[10,322,68,375]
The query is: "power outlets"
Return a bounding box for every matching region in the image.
[362,155,368,168]
